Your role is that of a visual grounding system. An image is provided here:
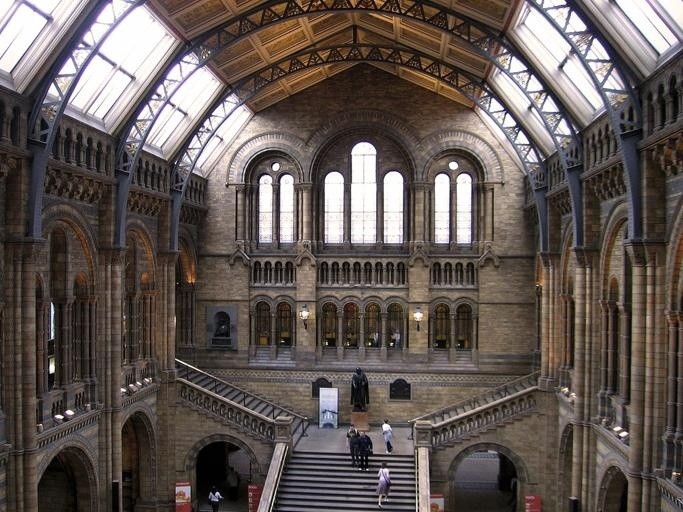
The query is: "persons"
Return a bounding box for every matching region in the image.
[209,486,225,511]
[347,424,360,467]
[382,418,393,452]
[377,463,391,509]
[351,367,370,412]
[358,431,373,472]
[226,466,241,503]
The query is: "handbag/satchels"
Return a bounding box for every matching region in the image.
[386,480,392,486]
[219,499,224,503]
[368,448,374,456]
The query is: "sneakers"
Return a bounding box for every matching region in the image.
[358,467,369,471]
